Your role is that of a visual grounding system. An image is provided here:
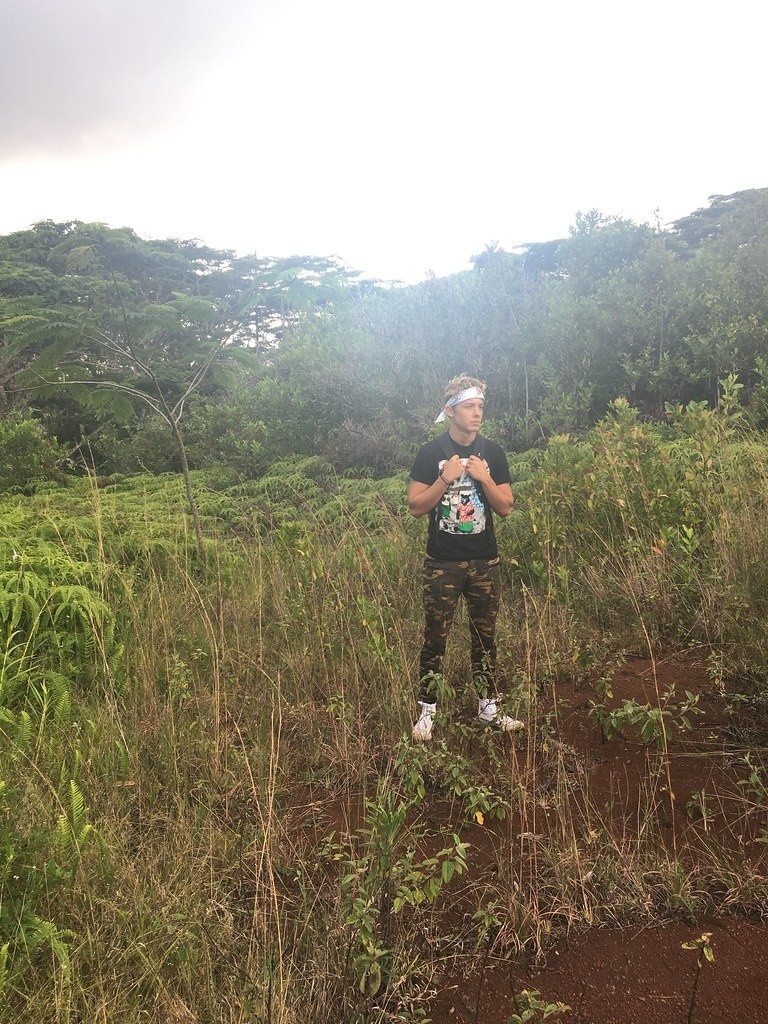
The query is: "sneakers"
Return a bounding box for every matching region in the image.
[479,708,524,732]
[412,703,437,740]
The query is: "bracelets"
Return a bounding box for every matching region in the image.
[440,474,450,485]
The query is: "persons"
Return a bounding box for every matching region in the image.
[407,372,524,740]
